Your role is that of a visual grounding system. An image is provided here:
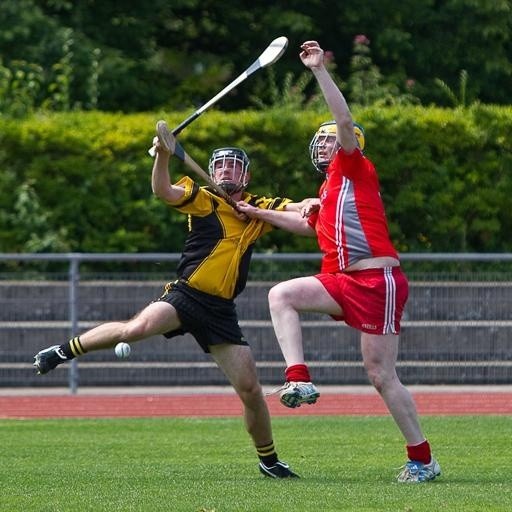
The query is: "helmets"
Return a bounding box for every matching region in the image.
[205,144,251,196]
[307,119,368,175]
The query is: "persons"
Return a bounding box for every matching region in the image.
[32,121,321,479]
[229,40,443,485]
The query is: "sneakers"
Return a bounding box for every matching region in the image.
[392,454,443,489]
[28,343,67,377]
[254,457,302,481]
[277,379,324,409]
